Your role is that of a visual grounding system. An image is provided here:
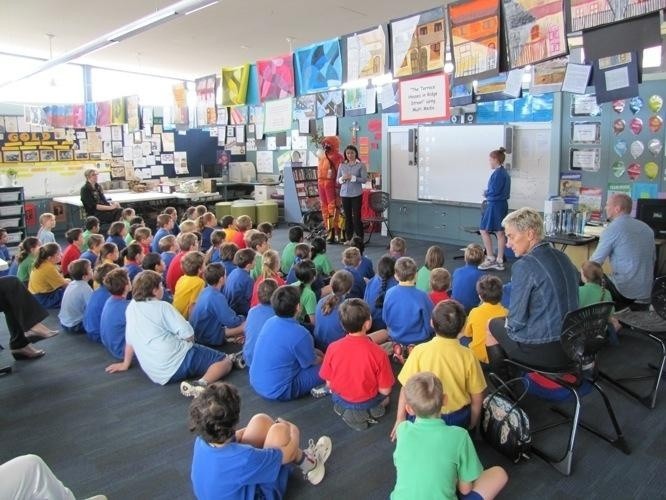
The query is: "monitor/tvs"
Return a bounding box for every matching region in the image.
[636,198,666,240]
[201,164,223,181]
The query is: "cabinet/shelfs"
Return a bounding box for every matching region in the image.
[0,186,27,260]
[282,164,323,227]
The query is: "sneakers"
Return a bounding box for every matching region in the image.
[332,402,387,432]
[389,341,422,364]
[227,343,253,370]
[476,257,506,271]
[304,435,332,485]
[303,378,341,398]
[178,376,215,401]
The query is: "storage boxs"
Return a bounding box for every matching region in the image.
[0,190,24,258]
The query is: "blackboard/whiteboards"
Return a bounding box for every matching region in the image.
[1,160,110,199]
[386,123,551,212]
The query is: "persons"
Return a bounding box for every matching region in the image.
[478,147,510,271]
[80,170,123,222]
[336,145,368,246]
[0,194,666,500]
[317,136,343,245]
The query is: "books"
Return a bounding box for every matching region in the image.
[292,168,323,213]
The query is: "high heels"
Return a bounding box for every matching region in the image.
[7,326,60,361]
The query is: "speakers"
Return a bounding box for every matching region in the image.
[450,115,460,124]
[408,129,415,165]
[465,112,475,125]
[503,127,514,174]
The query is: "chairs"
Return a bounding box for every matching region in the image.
[363,190,394,249]
[499,298,635,479]
[452,200,500,261]
[593,273,666,412]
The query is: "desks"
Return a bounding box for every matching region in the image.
[51,179,282,237]
[542,232,611,284]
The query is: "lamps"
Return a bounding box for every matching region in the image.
[16,1,221,81]
